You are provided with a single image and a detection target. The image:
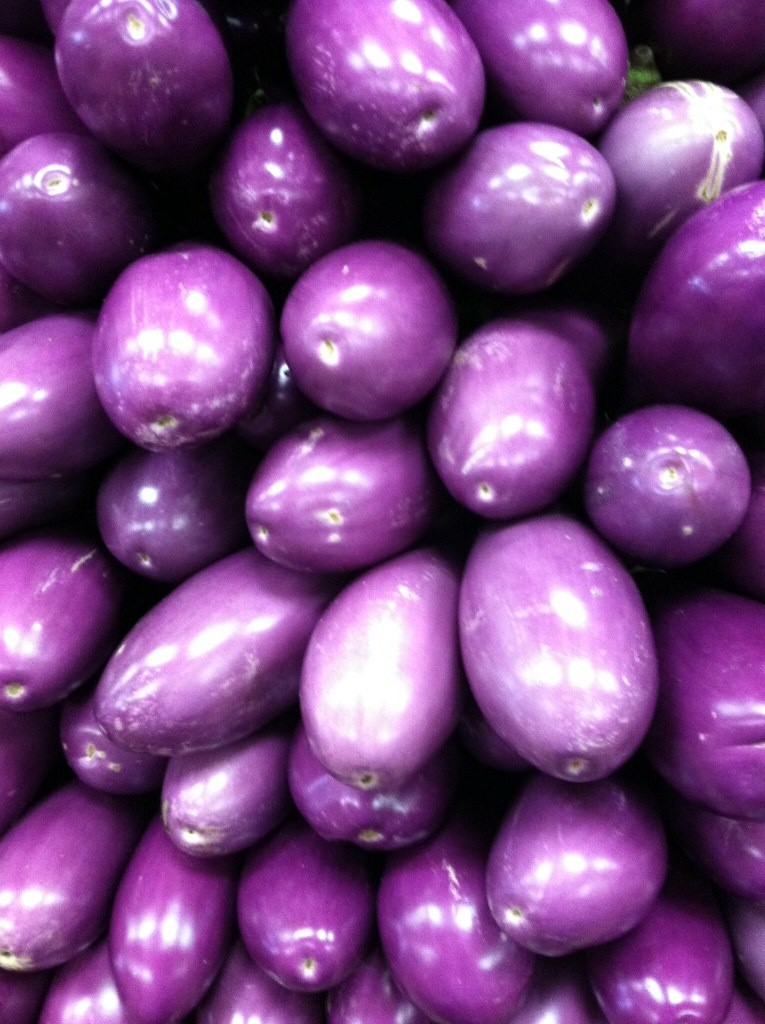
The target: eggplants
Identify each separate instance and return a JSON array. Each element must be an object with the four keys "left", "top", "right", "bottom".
[{"left": 0, "top": 0, "right": 765, "bottom": 1024}]
